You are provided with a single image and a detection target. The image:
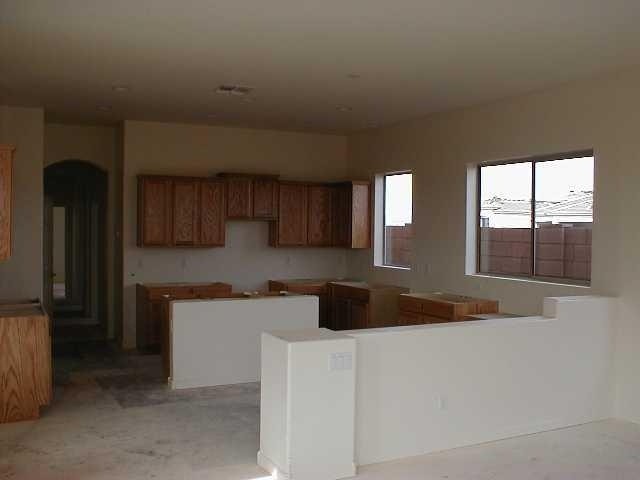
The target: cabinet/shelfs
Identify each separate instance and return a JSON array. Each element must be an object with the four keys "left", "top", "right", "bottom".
[
  {"left": 225, "top": 175, "right": 278, "bottom": 221},
  {"left": 0, "top": 143, "right": 16, "bottom": 261},
  {"left": 172, "top": 177, "right": 226, "bottom": 246},
  {"left": 400, "top": 293, "right": 498, "bottom": 325},
  {"left": 269, "top": 182, "right": 336, "bottom": 247},
  {"left": 135, "top": 283, "right": 232, "bottom": 354},
  {"left": 327, "top": 282, "right": 410, "bottom": 330},
  {"left": 336, "top": 181, "right": 371, "bottom": 248},
  {"left": 269, "top": 280, "right": 327, "bottom": 329},
  {"left": 136, "top": 174, "right": 170, "bottom": 247},
  {"left": 0, "top": 297, "right": 52, "bottom": 423}
]
